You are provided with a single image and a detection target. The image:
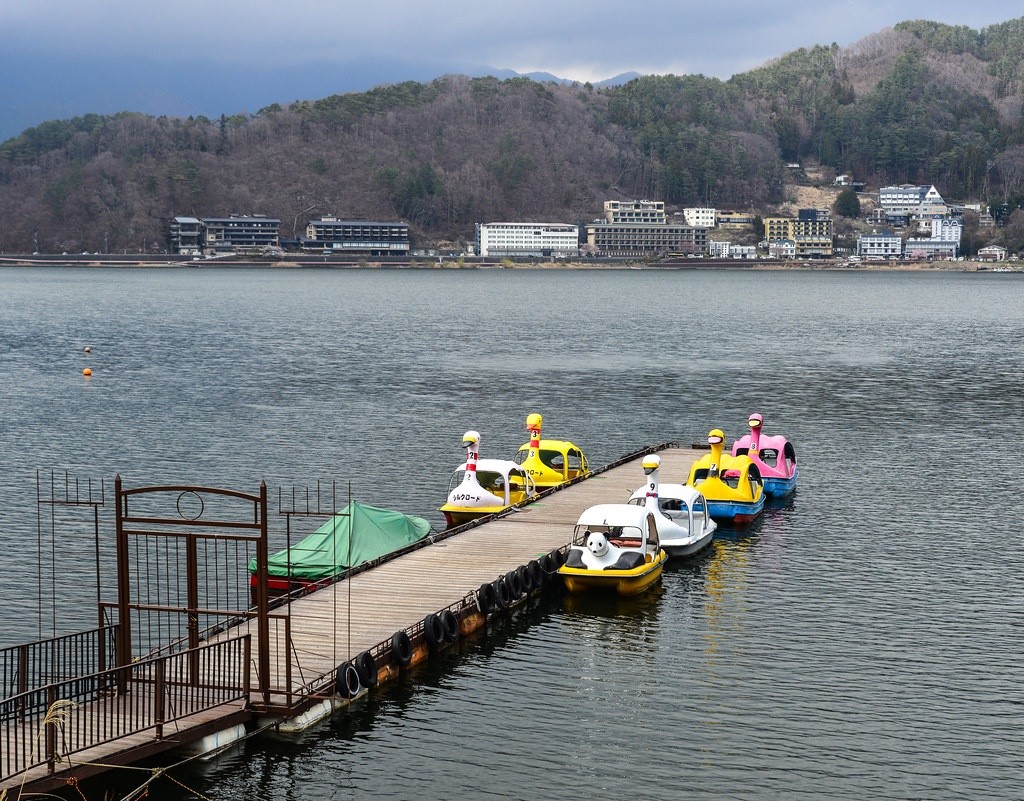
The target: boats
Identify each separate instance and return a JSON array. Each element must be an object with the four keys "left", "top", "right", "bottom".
[
  {"left": 731, "top": 413, "right": 800, "bottom": 496},
  {"left": 556, "top": 503, "right": 668, "bottom": 595},
  {"left": 436, "top": 430, "right": 540, "bottom": 525},
  {"left": 684, "top": 429, "right": 766, "bottom": 524},
  {"left": 248, "top": 513, "right": 431, "bottom": 603},
  {"left": 626, "top": 454, "right": 719, "bottom": 556},
  {"left": 509, "top": 413, "right": 589, "bottom": 489}
]
